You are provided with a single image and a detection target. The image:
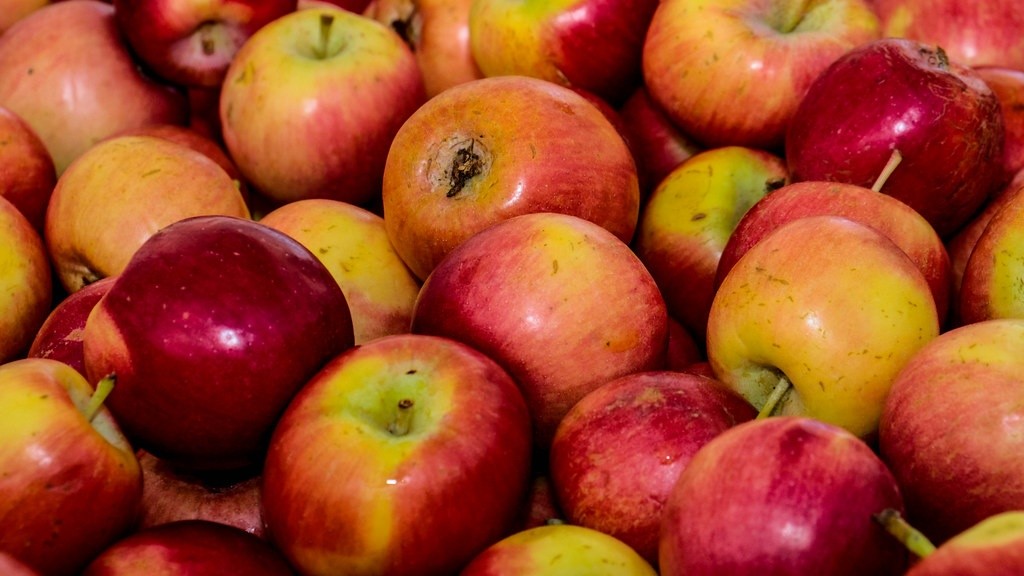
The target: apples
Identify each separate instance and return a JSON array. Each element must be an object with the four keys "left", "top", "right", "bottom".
[{"left": 0, "top": 0, "right": 1024, "bottom": 576}]
